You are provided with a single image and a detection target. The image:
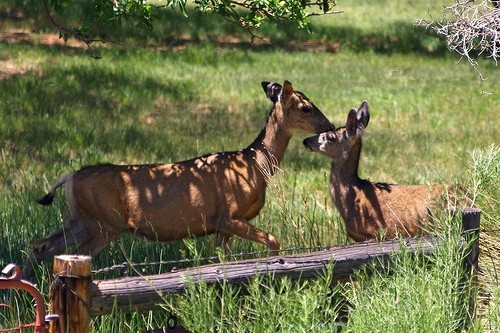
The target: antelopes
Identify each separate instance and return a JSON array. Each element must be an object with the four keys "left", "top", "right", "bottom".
[
  {"left": 303, "top": 101, "right": 492, "bottom": 305},
  {"left": 18, "top": 80, "right": 336, "bottom": 281}
]
